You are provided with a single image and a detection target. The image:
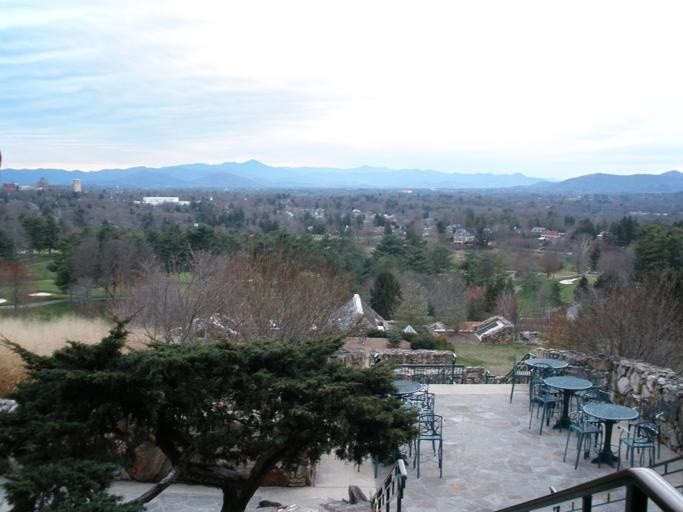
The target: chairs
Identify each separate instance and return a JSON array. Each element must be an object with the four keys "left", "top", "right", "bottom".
[
  {"left": 510, "top": 347, "right": 682, "bottom": 472},
  {"left": 353, "top": 374, "right": 446, "bottom": 485}
]
